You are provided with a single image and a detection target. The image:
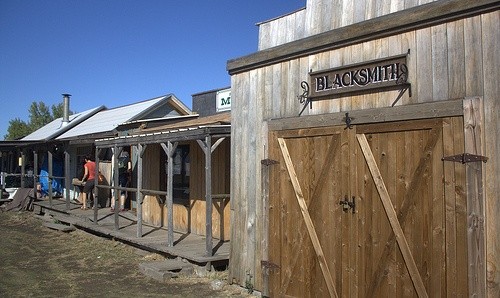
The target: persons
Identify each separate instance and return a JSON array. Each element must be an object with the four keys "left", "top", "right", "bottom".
[
  {"left": 81, "top": 153, "right": 100, "bottom": 209},
  {"left": 110, "top": 156, "right": 132, "bottom": 211}
]
